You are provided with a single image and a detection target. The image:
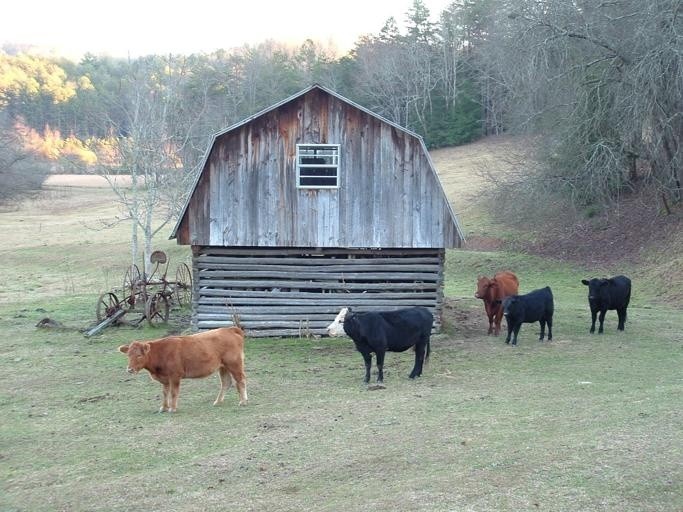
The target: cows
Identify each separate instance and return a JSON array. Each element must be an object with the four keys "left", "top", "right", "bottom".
[
  {"left": 494, "top": 286, "right": 554, "bottom": 346},
  {"left": 475, "top": 271, "right": 520, "bottom": 337},
  {"left": 322, "top": 306, "right": 435, "bottom": 383},
  {"left": 579, "top": 273, "right": 633, "bottom": 335},
  {"left": 116, "top": 312, "right": 249, "bottom": 414}
]
